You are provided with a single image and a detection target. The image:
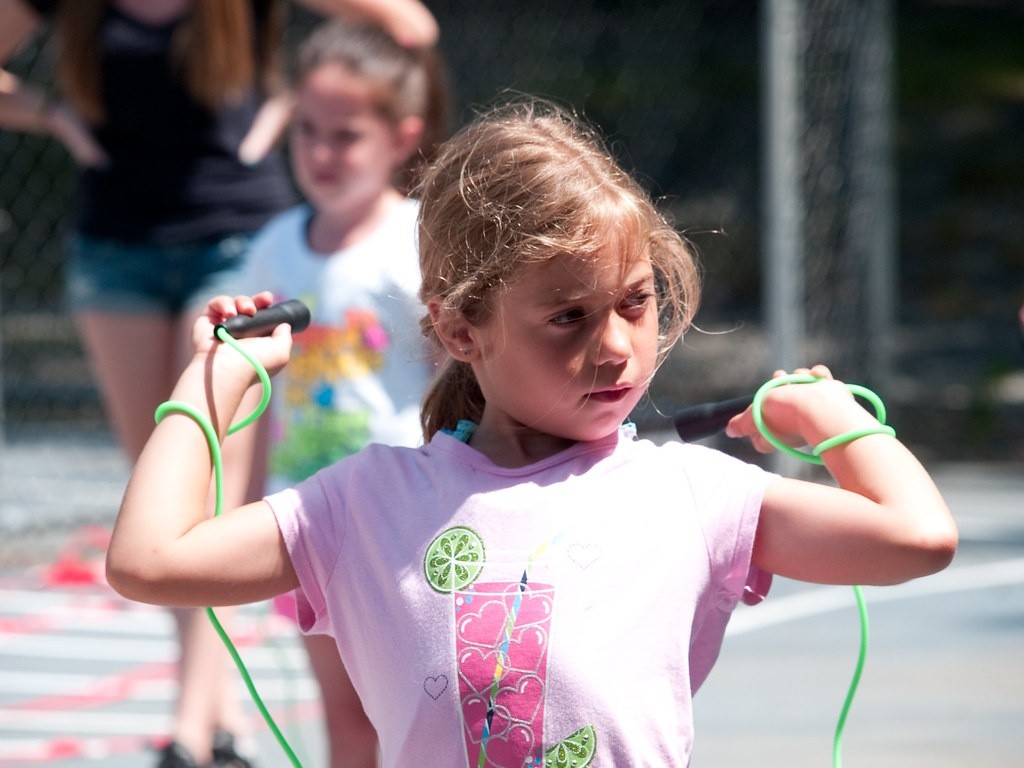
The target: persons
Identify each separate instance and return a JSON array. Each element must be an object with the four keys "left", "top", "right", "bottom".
[
  {"left": 178, "top": 22, "right": 449, "bottom": 768},
  {"left": 0, "top": 0, "right": 439, "bottom": 768},
  {"left": 105, "top": 115, "right": 960, "bottom": 768}
]
